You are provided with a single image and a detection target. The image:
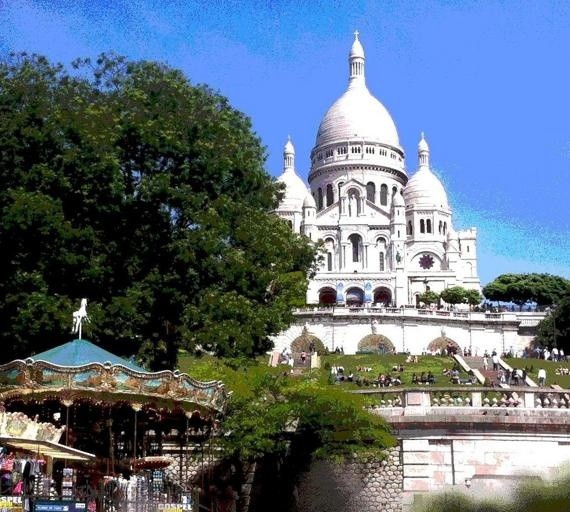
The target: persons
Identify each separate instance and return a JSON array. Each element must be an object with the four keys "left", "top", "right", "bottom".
[{"left": 277, "top": 338, "right": 567, "bottom": 387}]
